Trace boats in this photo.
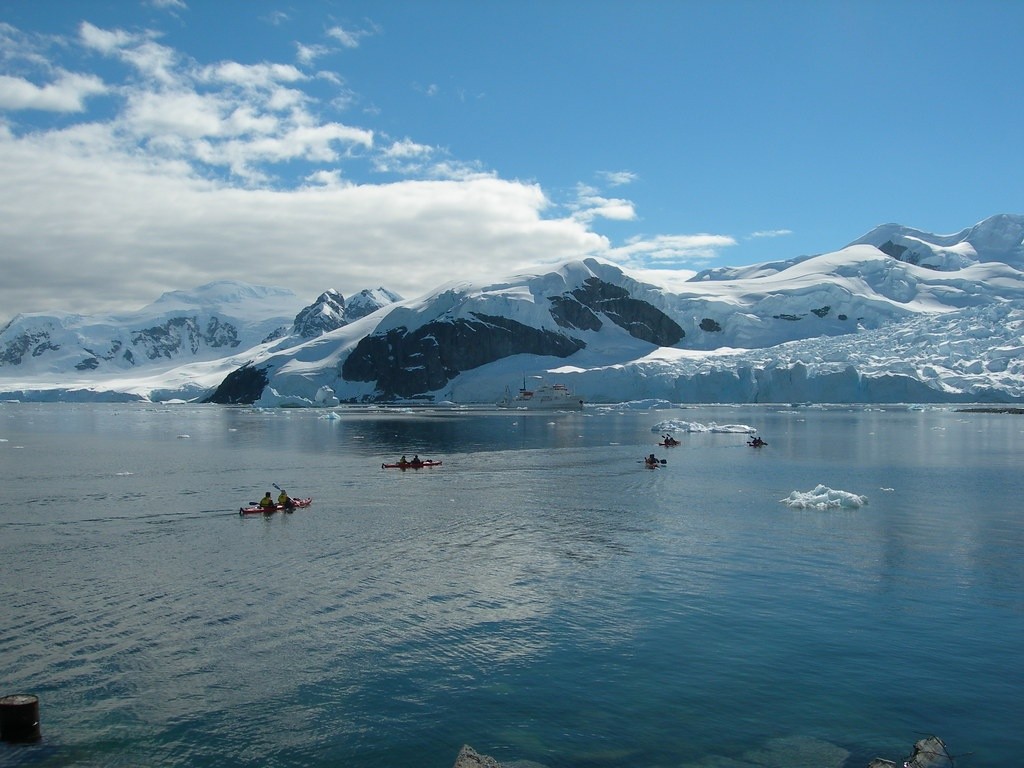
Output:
[383,460,443,469]
[749,440,766,446]
[645,457,657,470]
[240,498,312,513]
[496,373,583,411]
[660,439,680,446]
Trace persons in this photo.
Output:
[414,455,420,461]
[400,456,408,464]
[664,437,675,444]
[278,490,289,504]
[260,492,273,506]
[752,437,763,443]
[645,454,659,463]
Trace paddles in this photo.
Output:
[667,433,676,443]
[661,435,665,438]
[248,502,260,505]
[272,482,300,507]
[750,435,768,445]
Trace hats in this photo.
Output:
[415,455,418,457]
[402,456,406,458]
